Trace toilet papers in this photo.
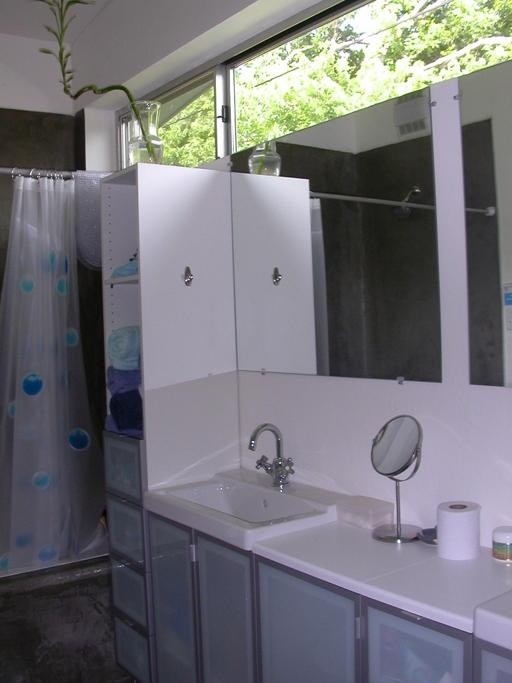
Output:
[436,499,482,561]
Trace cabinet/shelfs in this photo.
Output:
[235,171,317,382]
[253,558,472,683]
[141,512,254,683]
[98,163,230,683]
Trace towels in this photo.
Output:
[106,323,144,437]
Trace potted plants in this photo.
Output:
[39,0,165,163]
[249,139,281,176]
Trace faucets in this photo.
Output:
[248,422,295,487]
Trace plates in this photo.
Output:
[416,526,436,546]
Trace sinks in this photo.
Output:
[141,475,339,553]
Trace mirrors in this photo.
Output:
[371,412,429,544]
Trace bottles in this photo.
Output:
[491,525,511,563]
